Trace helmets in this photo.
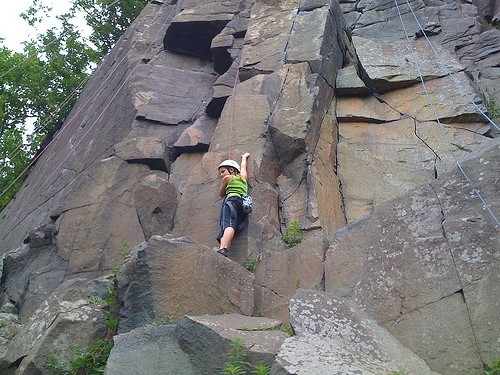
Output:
[218,159,240,173]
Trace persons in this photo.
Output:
[211,152,250,258]
[26,120,64,170]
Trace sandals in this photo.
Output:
[216,248,228,258]
